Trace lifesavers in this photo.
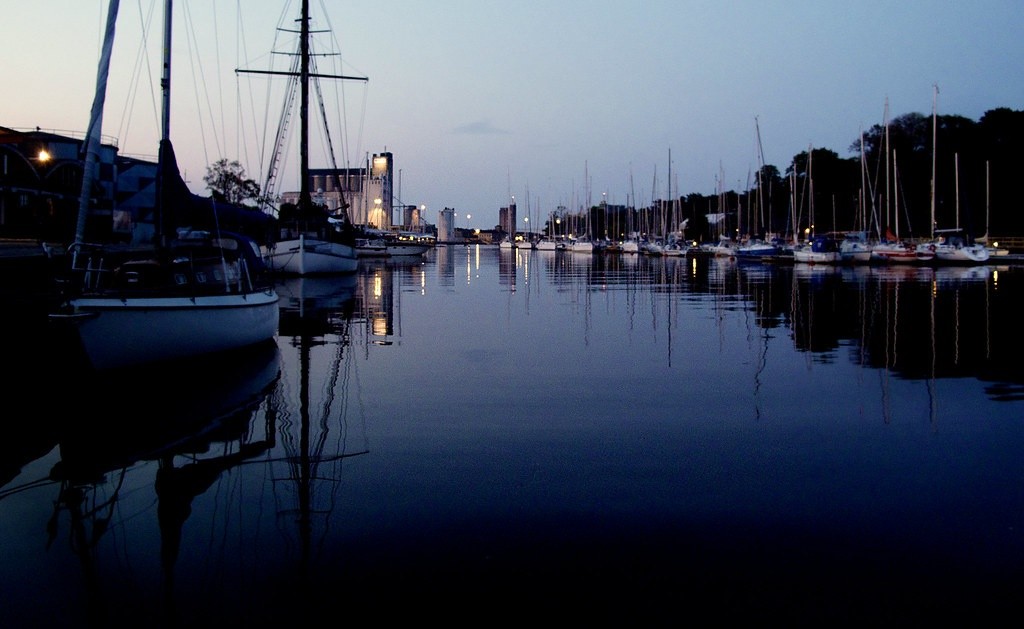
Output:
[909,243,917,251]
[929,244,937,252]
[46,251,87,287]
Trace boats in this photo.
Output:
[22,335,288,629]
[271,271,363,629]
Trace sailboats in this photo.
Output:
[497,82,1010,266]
[1,0,440,374]
